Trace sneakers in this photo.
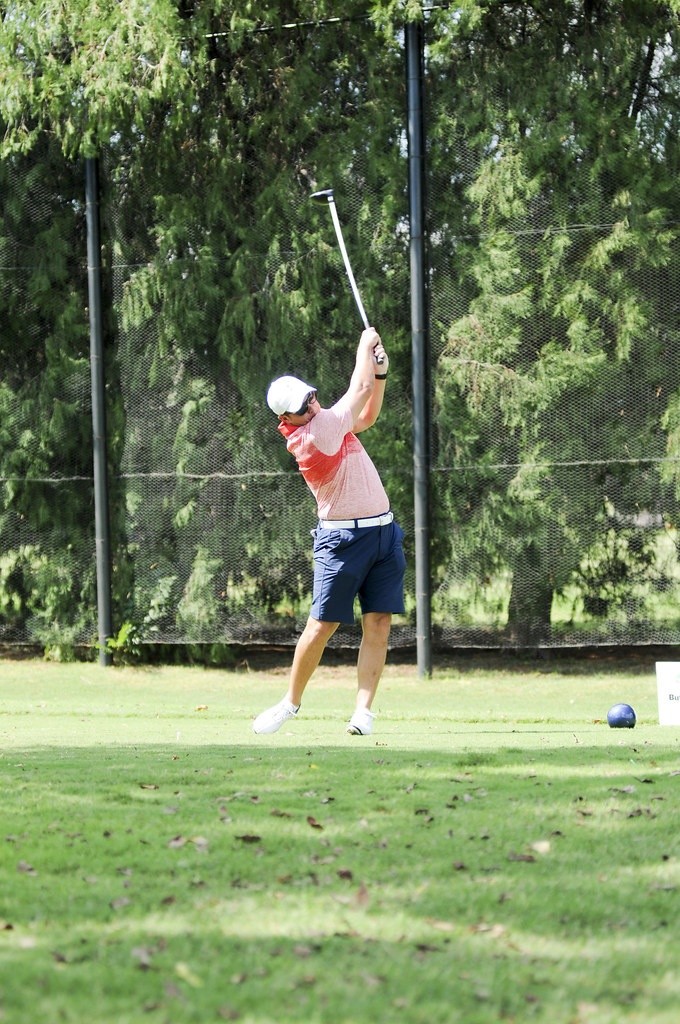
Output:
[251,692,301,734]
[345,706,376,735]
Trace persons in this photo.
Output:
[255,325,407,737]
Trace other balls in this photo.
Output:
[607,704,635,729]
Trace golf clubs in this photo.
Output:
[307,189,385,366]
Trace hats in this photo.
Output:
[267,375,318,416]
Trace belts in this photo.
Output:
[320,512,394,529]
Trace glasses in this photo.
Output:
[285,391,317,416]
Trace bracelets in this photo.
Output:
[376,372,388,379]
